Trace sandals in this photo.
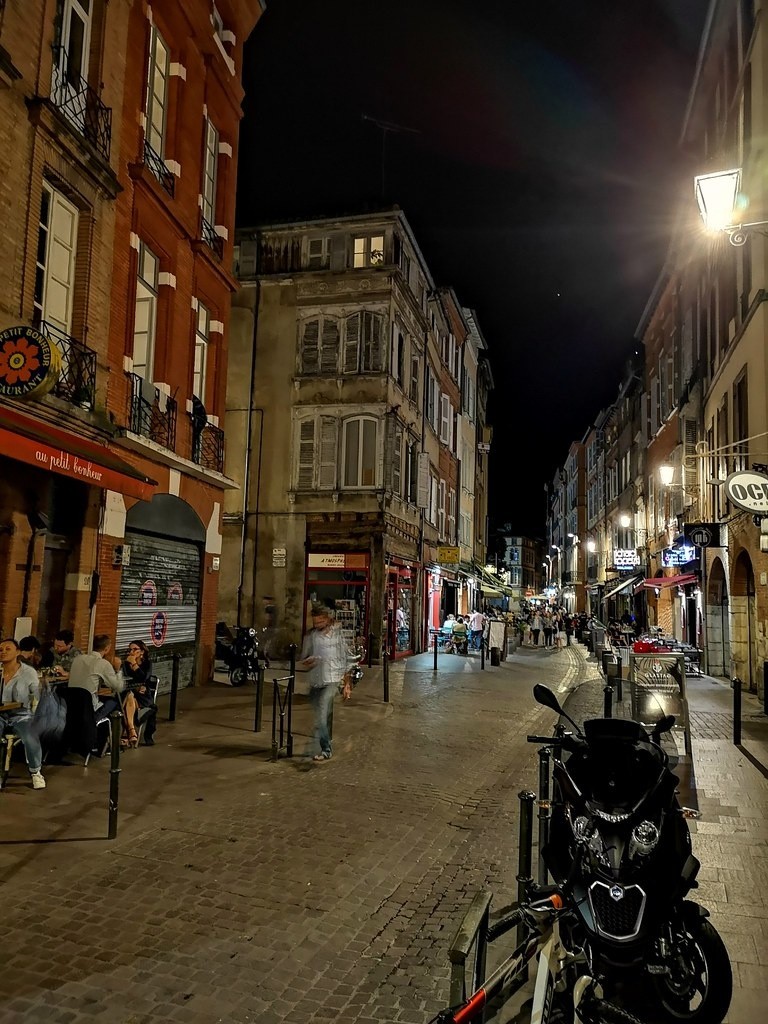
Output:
[119,729,137,750]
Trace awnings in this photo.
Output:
[644,575,697,589]
[603,577,638,599]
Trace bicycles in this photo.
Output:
[425,871,651,1024]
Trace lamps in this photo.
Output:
[688,152,768,248]
[658,458,701,498]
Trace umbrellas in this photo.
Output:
[482,586,503,598]
[529,596,548,610]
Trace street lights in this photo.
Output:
[551,544,561,590]
[543,563,548,586]
[568,533,578,582]
[546,555,552,584]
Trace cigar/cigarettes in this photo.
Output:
[348,697,350,698]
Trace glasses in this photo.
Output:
[126,648,142,652]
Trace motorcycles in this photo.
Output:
[526,678,733,1024]
[214,622,267,688]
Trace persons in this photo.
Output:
[527,601,574,652]
[0,629,153,789]
[443,606,505,654]
[300,607,352,761]
[396,606,406,627]
[572,610,597,644]
[607,608,637,648]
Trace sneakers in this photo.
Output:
[31,772,46,789]
[314,746,333,760]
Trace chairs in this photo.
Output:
[42,687,112,766]
[429,628,471,654]
[1,734,20,788]
[134,675,159,750]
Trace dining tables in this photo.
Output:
[98,685,113,696]
[44,675,68,684]
[0,699,23,711]
